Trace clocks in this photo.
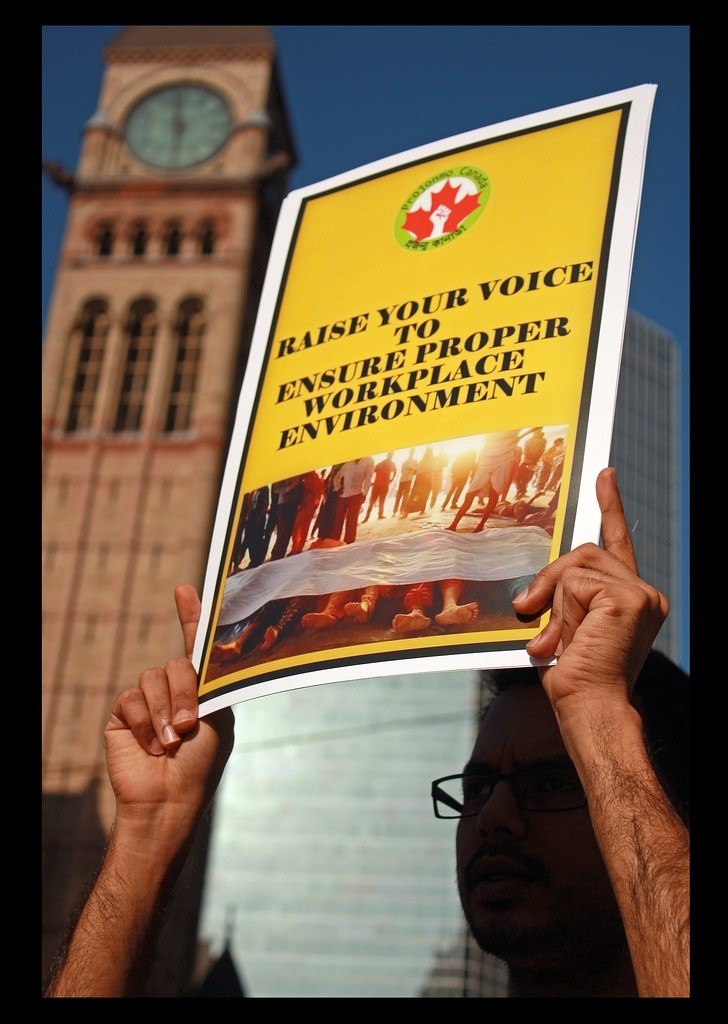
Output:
[120,83,233,170]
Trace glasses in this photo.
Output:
[432,758,587,818]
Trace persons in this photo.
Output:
[41,467,691,998]
[209,428,565,660]
